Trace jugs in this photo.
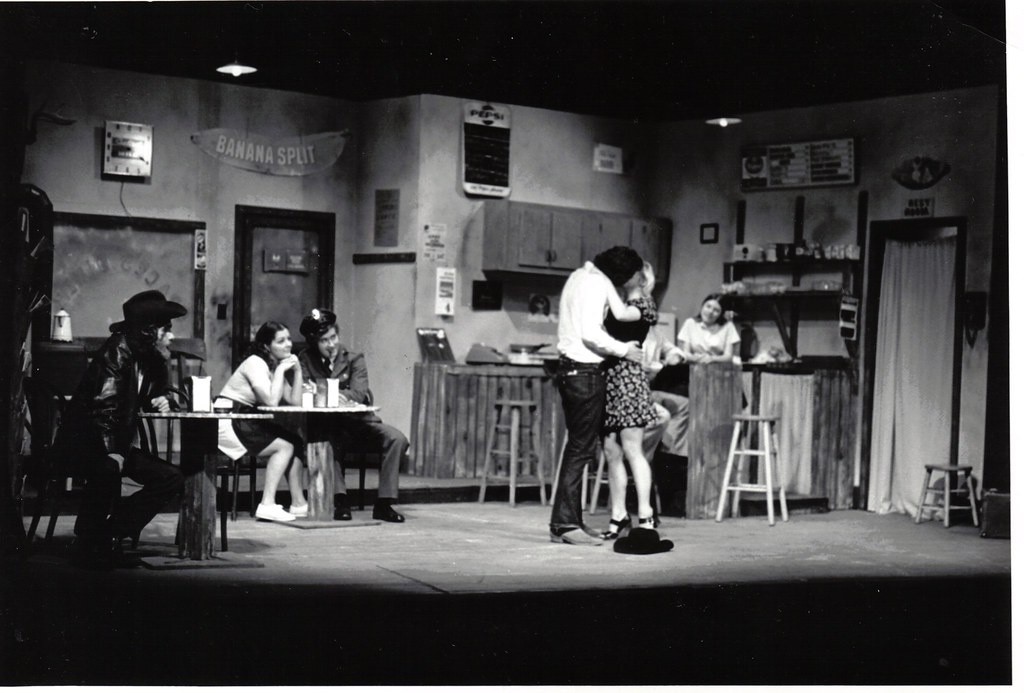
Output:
[52,308,73,343]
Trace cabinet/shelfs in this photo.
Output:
[465,197,674,282]
[407,373,566,485]
[734,365,859,510]
[719,257,860,357]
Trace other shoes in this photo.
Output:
[551,523,605,546]
[67,538,133,570]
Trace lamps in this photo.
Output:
[215,49,258,78]
[704,108,743,129]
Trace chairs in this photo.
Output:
[136,386,230,553]
[183,376,258,520]
[21,373,126,560]
[340,390,379,512]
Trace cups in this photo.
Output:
[316,378,325,407]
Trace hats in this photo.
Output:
[614,527,675,554]
[299,308,339,337]
[110,290,188,332]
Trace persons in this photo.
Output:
[46,289,188,571]
[279,309,409,524]
[548,245,690,548]
[213,320,309,523]
[677,293,748,410]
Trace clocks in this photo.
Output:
[101,117,154,178]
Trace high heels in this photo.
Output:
[638,508,662,528]
[600,512,633,541]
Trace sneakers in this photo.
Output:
[289,501,308,517]
[256,502,295,523]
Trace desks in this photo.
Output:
[256,404,383,530]
[136,411,275,571]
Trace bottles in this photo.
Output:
[301,384,314,408]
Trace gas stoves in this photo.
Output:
[509,351,558,364]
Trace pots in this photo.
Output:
[510,343,551,352]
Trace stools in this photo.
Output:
[477,397,547,508]
[715,412,791,528]
[915,461,979,529]
[588,431,662,517]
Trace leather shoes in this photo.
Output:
[334,504,351,519]
[372,506,404,522]
